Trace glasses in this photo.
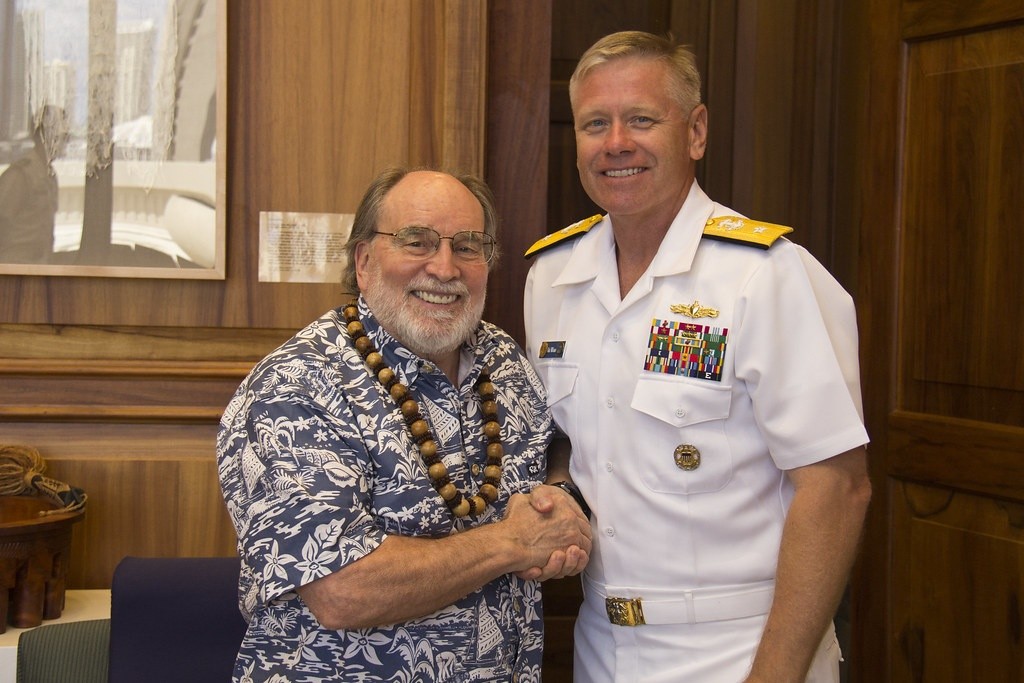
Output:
[368,226,497,264]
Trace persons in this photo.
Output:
[216,165,595,683]
[522,30,875,683]
[0,105,70,265]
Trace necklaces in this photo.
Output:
[343,299,504,516]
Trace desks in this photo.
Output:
[0,492,87,635]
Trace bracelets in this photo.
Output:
[550,480,592,522]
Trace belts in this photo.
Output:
[581,577,775,627]
